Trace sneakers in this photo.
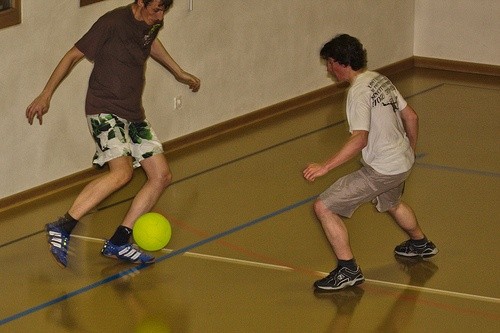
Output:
[394,239,438,256]
[101,239,156,264]
[45,216,70,268]
[314,264,365,290]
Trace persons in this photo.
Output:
[26,0,201,268]
[302,33,439,290]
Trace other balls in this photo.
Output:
[134,213,170,250]
